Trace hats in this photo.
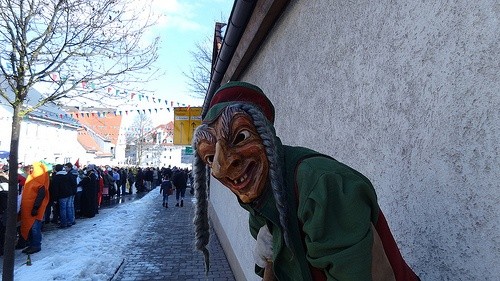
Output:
[201,81,275,125]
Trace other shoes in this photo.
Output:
[162,203,168,208]
[175,203,179,207]
[181,200,183,207]
[22,246,41,254]
[44,215,76,228]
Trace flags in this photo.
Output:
[74,159,81,168]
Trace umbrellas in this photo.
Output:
[0,151,10,159]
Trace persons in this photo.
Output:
[194,81,423,281]
[160,175,172,208]
[172,169,188,207]
[0,161,188,255]
